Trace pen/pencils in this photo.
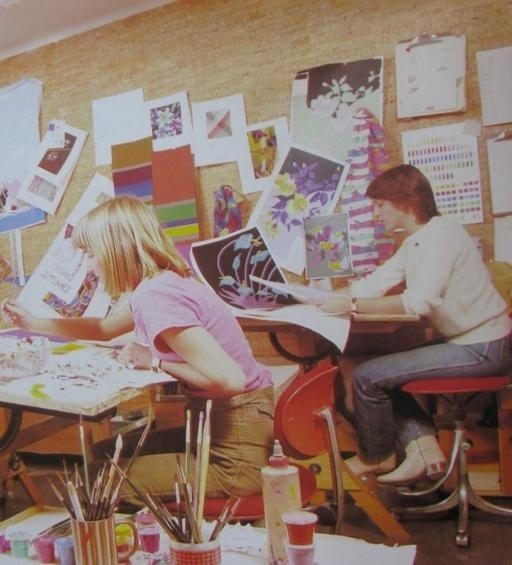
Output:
[3,291,13,310]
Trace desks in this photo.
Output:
[1,507,419,565]
[1,327,160,507]
[232,312,420,544]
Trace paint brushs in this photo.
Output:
[46,409,152,520]
[104,400,241,544]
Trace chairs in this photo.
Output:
[390,374,509,544]
[166,363,343,533]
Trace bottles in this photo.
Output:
[259,438,302,565]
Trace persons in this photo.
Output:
[2,195,280,515]
[287,163,510,487]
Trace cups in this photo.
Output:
[68,510,139,565]
[53,538,75,565]
[32,535,56,563]
[167,528,223,565]
[280,511,318,545]
[284,539,316,565]
[134,513,161,553]
[9,532,30,558]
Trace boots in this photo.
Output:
[378,435,446,484]
[344,452,396,476]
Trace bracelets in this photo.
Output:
[351,297,359,313]
